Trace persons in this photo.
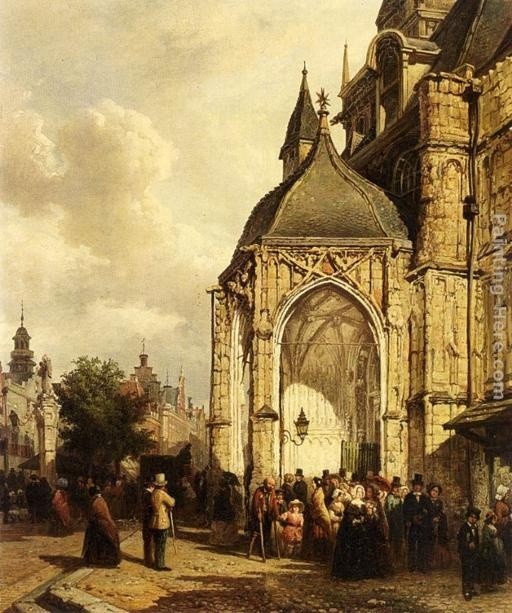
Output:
[0,444,512,602]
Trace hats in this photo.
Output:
[426,481,441,495]
[287,497,305,512]
[464,507,482,521]
[412,473,424,486]
[151,470,168,486]
[390,474,405,488]
[294,467,386,486]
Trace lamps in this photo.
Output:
[278,406,310,445]
